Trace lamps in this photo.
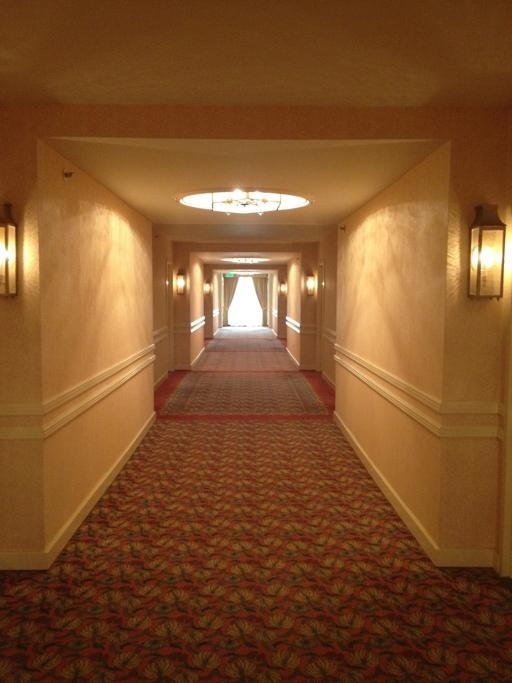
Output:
[175,267,186,295]
[465,201,506,299]
[173,187,313,214]
[204,279,211,295]
[306,272,317,296]
[280,281,286,294]
[0,201,20,298]
